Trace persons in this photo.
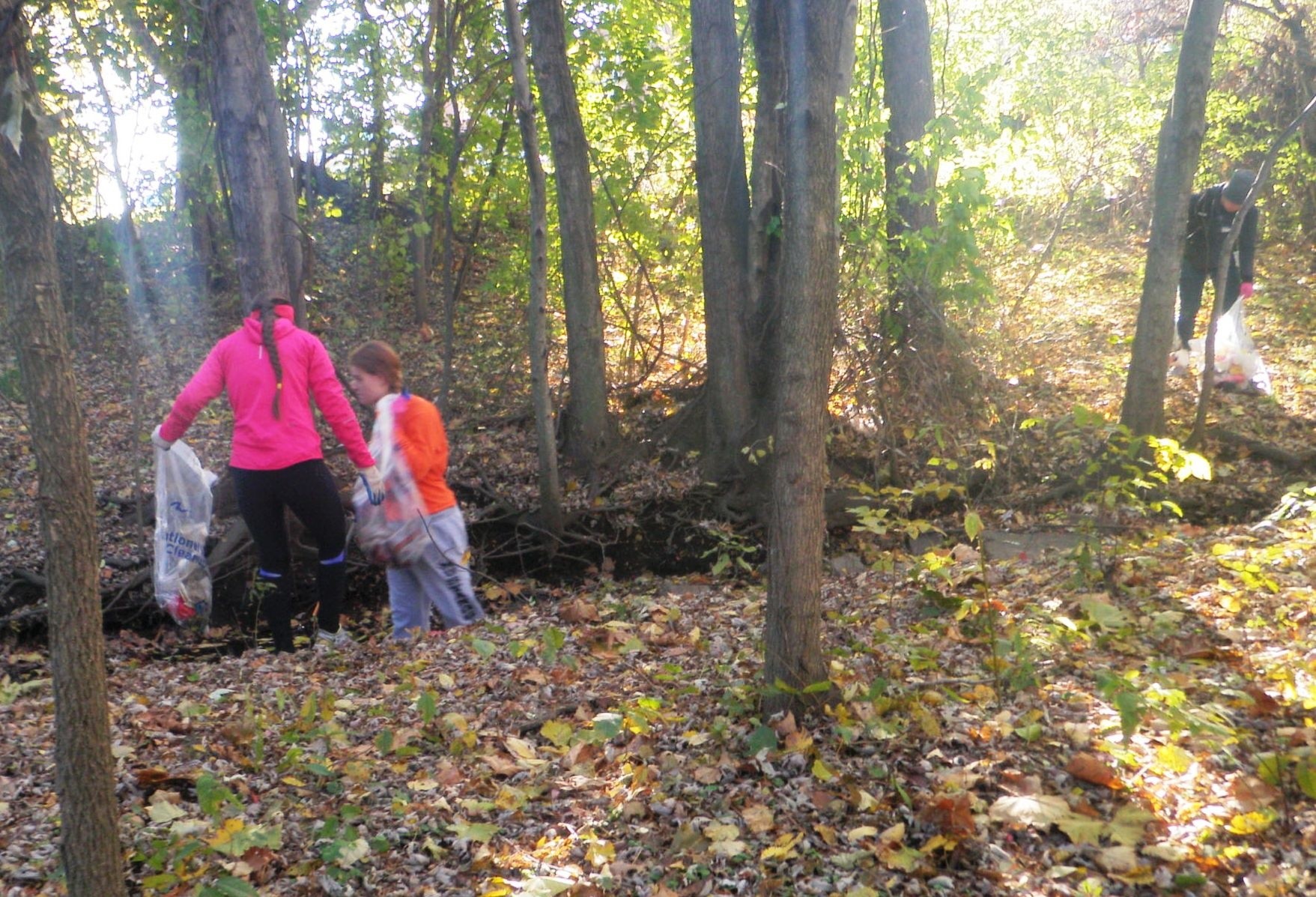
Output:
[151,288,386,657]
[346,339,487,642]
[1177,170,1258,353]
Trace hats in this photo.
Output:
[1223,166,1255,204]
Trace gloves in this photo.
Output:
[354,465,385,505]
[151,424,176,451]
[1239,280,1253,298]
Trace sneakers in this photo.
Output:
[313,622,359,652]
